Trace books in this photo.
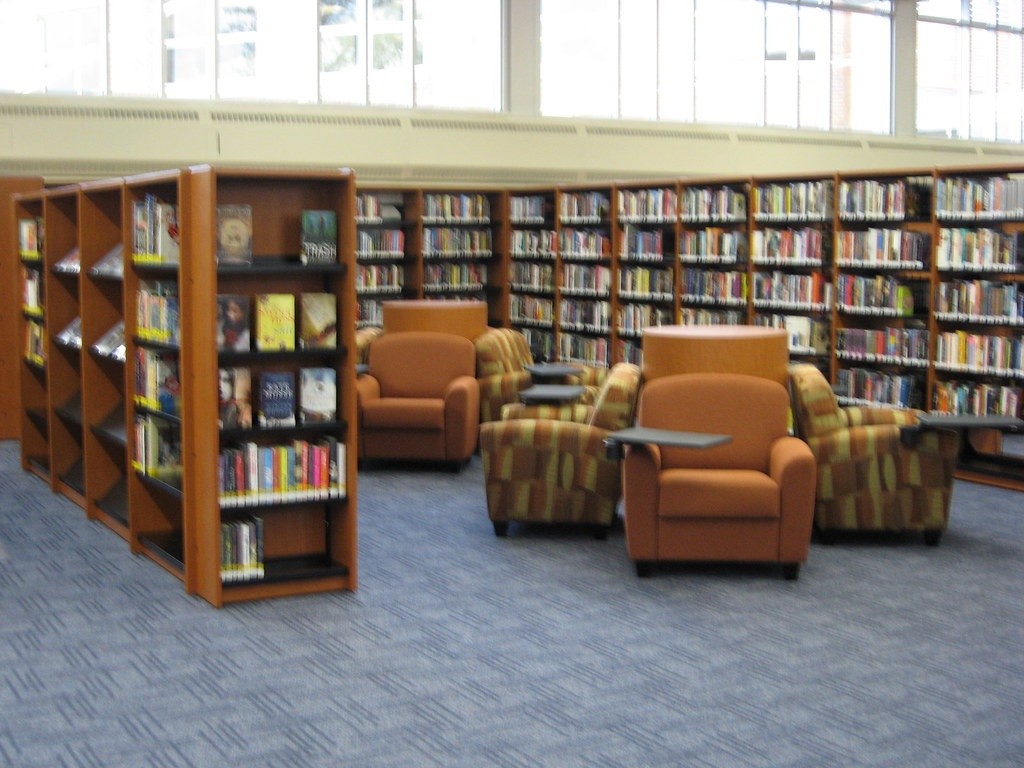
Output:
[257,372,296,428]
[216,294,251,351]
[217,367,253,431]
[300,209,338,263]
[299,292,337,350]
[55,244,125,362]
[220,437,346,583]
[215,204,252,265]
[132,196,183,477]
[255,293,296,352]
[356,172,1024,417]
[19,217,44,364]
[299,368,336,425]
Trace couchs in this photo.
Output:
[478,362,643,540]
[475,325,607,423]
[789,361,961,549]
[602,370,819,582]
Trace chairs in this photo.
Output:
[356,330,481,476]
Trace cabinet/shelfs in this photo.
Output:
[509,162,1024,490]
[0,165,359,608]
[355,182,510,334]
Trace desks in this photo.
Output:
[383,301,491,337]
[642,324,790,384]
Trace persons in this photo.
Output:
[219,297,250,349]
[218,369,242,430]
